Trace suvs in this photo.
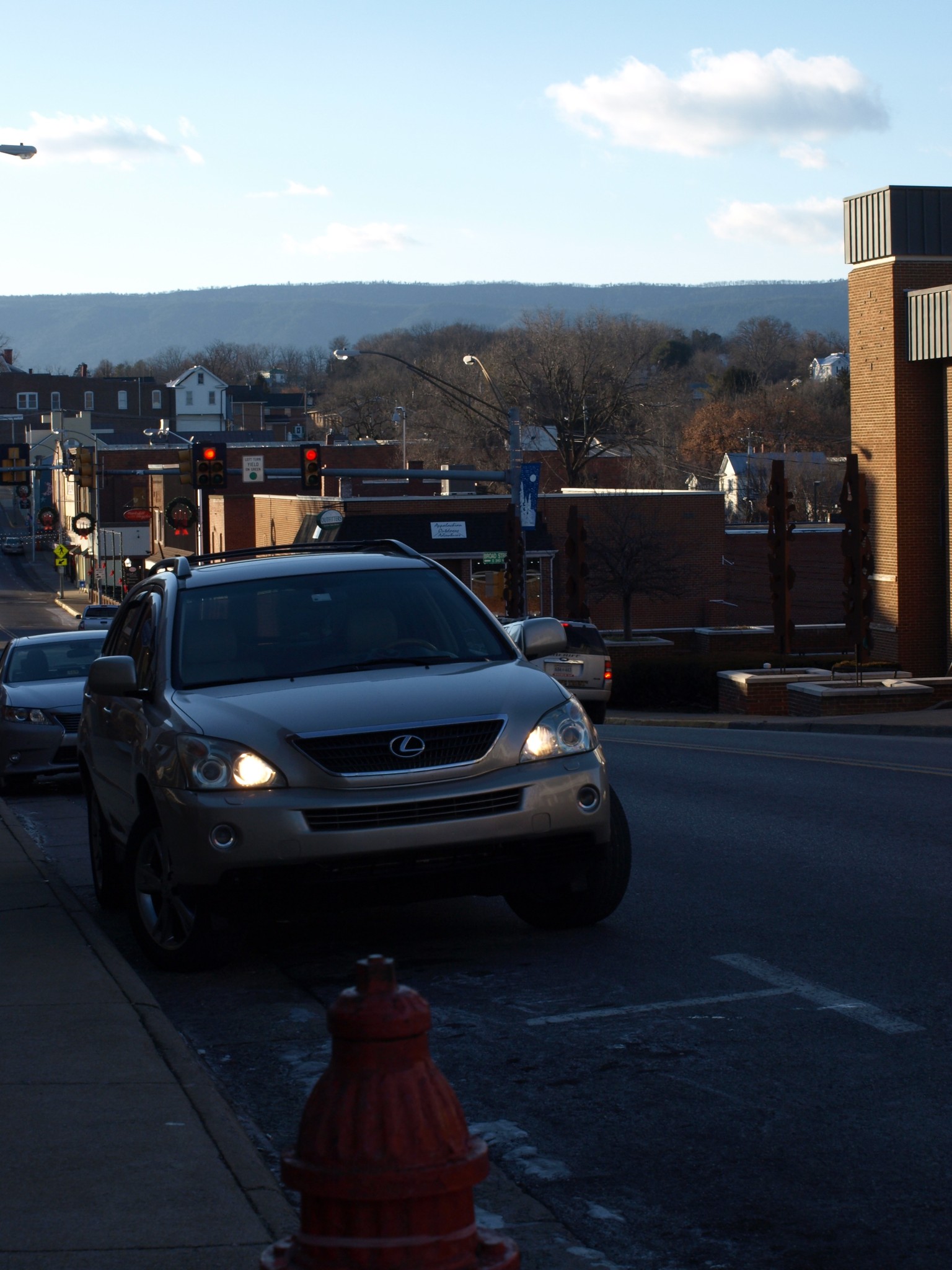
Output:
[502,618,613,727]
[68,533,631,983]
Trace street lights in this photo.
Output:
[814,479,821,521]
[392,402,408,476]
[332,346,524,613]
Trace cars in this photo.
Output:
[1,536,24,555]
[0,630,116,796]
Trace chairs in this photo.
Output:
[181,616,269,686]
[328,604,405,666]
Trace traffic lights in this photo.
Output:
[191,440,229,491]
[73,451,93,487]
[301,442,322,489]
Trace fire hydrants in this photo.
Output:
[252,950,528,1270]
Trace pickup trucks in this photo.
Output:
[75,604,121,632]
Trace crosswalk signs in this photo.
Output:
[54,543,68,559]
